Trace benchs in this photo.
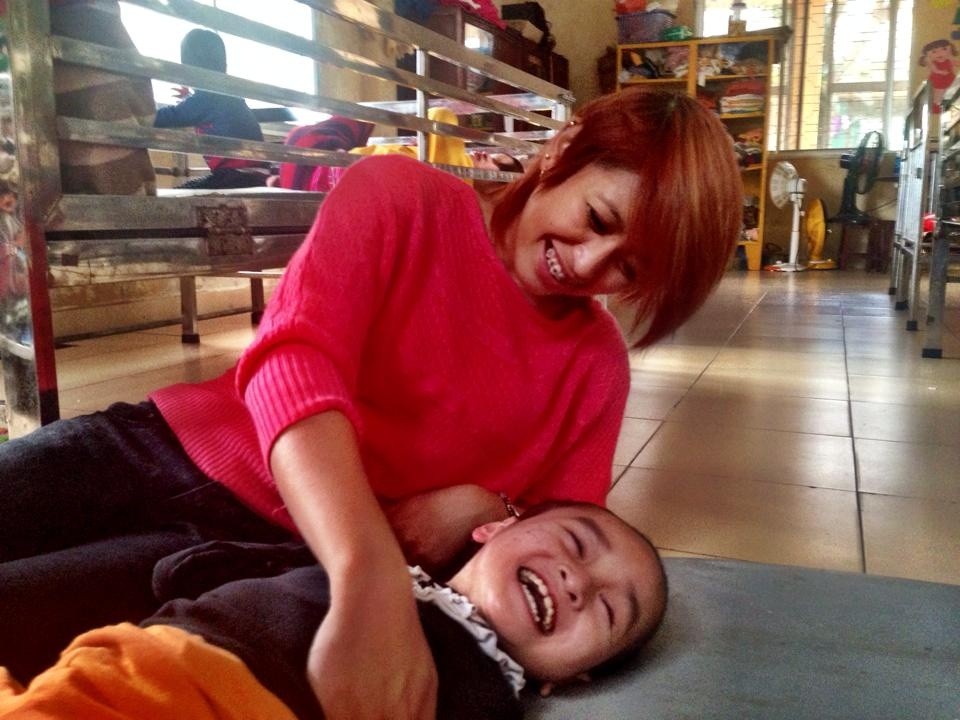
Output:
[0,0,577,427]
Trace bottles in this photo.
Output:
[728,0,747,37]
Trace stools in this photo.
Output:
[838,220,897,273]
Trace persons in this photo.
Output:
[0,0,156,194]
[0,86,745,690]
[0,499,671,719]
[154,29,269,189]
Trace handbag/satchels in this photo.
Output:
[501,2,552,47]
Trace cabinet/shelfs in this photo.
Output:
[617,31,781,271]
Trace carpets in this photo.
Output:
[528,556,960,720]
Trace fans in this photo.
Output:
[828,131,884,224]
[762,158,811,271]
[802,198,837,270]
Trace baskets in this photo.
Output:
[615,9,678,45]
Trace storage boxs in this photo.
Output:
[614,10,677,44]
